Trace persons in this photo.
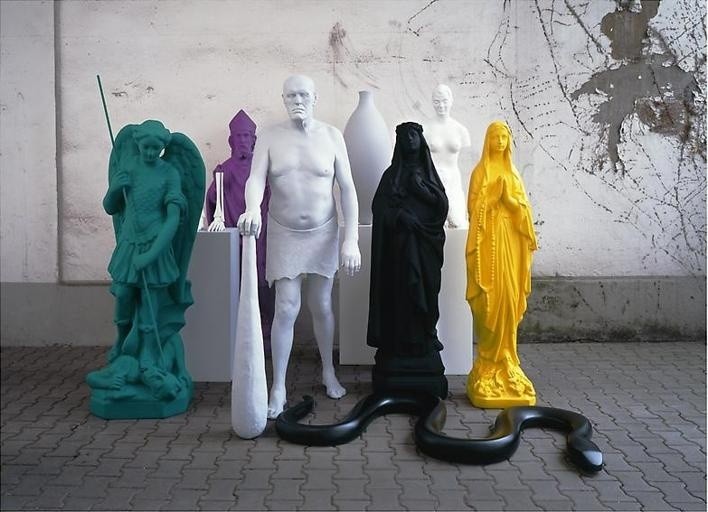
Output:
[461,119,540,410]
[423,82,464,228]
[84,119,207,385]
[235,73,364,422]
[205,108,278,358]
[80,353,183,402]
[366,120,450,389]
